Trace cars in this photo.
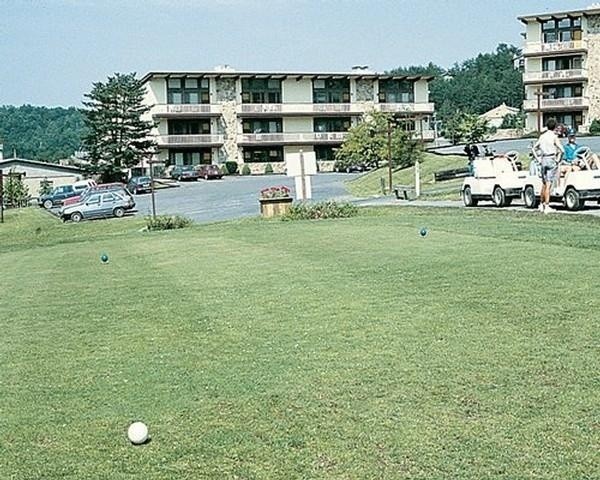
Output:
[333,155,364,173]
[34,161,226,224]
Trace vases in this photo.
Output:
[258,198,293,219]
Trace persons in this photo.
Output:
[531,119,564,215]
[560,129,578,164]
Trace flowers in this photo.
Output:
[261,184,290,198]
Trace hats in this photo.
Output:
[566,128,577,136]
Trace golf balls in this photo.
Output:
[126,421,148,444]
[420,230,427,235]
[102,255,108,262]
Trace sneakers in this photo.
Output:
[537,202,556,214]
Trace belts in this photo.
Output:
[542,153,555,157]
